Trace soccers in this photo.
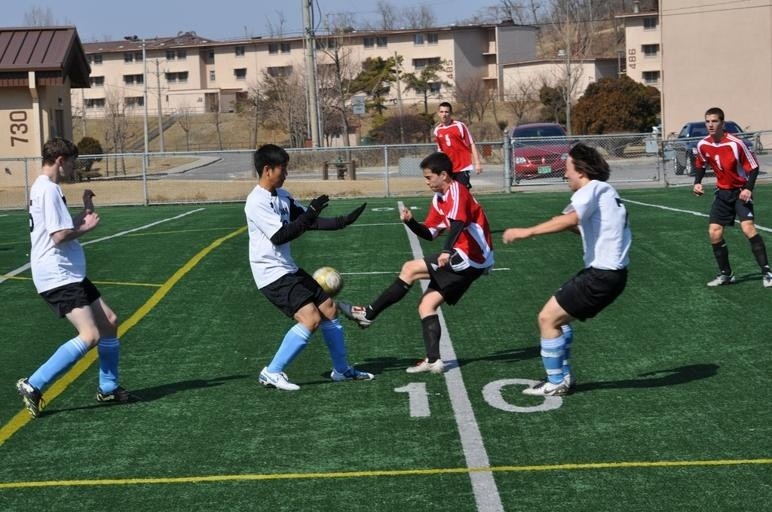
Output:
[313,266,342,297]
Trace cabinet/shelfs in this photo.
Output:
[323,160,355,180]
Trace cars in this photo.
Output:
[509,123,577,183]
[671,120,754,177]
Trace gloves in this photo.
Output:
[344,203,366,225]
[305,195,329,221]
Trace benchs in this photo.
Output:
[622,143,664,159]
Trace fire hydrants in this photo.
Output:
[334,157,348,181]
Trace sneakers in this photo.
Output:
[706,272,736,286]
[330,366,374,381]
[339,301,374,329]
[406,358,445,375]
[258,367,300,390]
[762,271,771,286]
[17,378,46,419]
[96,386,142,402]
[522,374,572,394]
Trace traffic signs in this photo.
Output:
[352,95,365,114]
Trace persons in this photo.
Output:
[337,153,497,375]
[14,138,143,418]
[244,144,375,391]
[500,143,634,397]
[693,106,772,288]
[432,101,483,191]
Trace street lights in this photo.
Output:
[557,49,573,137]
[122,32,147,161]
